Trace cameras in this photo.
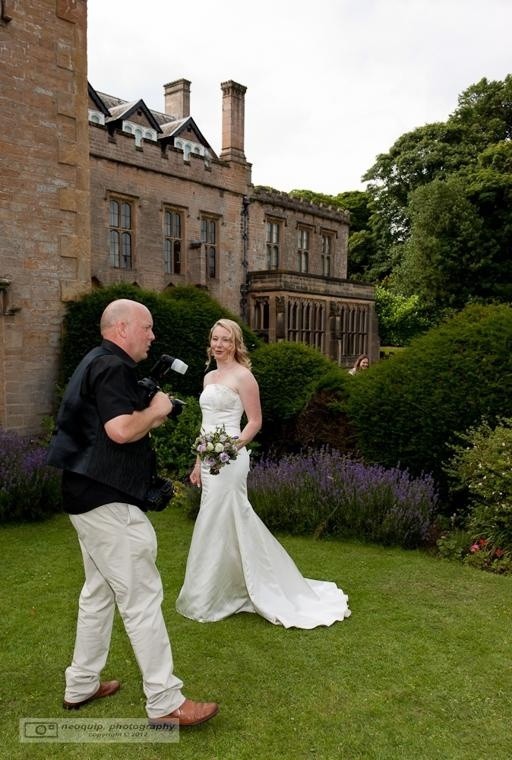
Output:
[138,353,189,421]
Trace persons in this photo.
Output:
[176,317,352,632]
[346,353,370,376]
[46,298,219,728]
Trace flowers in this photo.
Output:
[193,424,239,473]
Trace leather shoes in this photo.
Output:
[148,699,218,727]
[64,680,119,709]
